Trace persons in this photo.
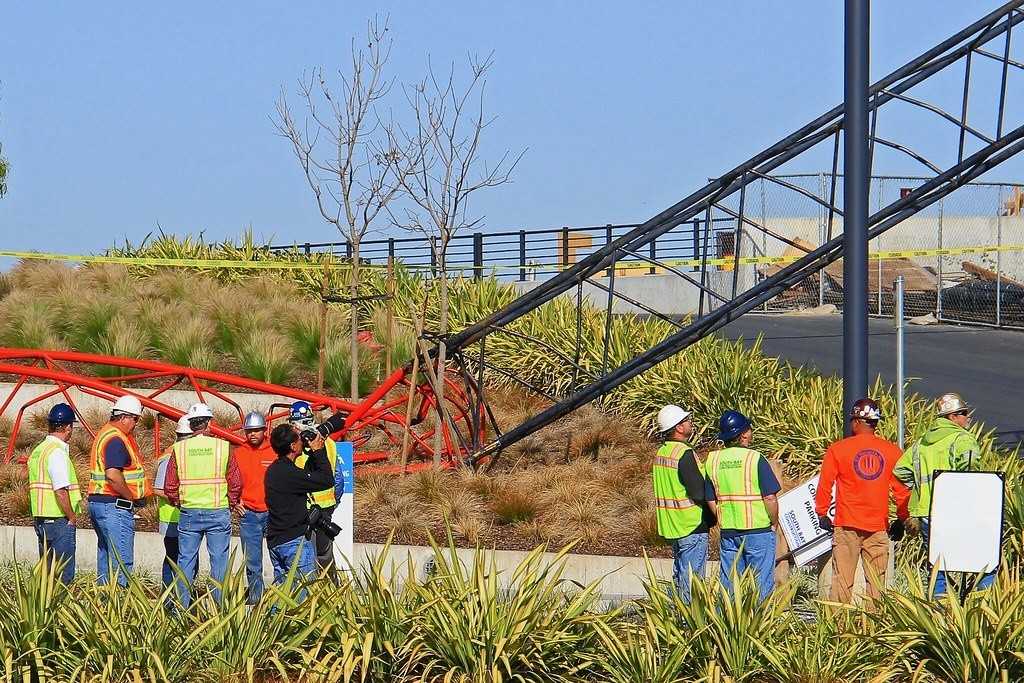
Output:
[234,412,278,618]
[27,403,82,601]
[286,402,344,589]
[705,411,782,626]
[892,394,997,619]
[652,404,717,624]
[263,424,336,632]
[815,398,913,636]
[153,414,198,612]
[164,404,243,618]
[87,394,153,607]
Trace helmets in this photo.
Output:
[243,412,266,429]
[175,415,194,433]
[934,393,969,415]
[657,405,691,432]
[286,401,314,420]
[110,395,142,416]
[45,403,79,423]
[850,398,882,419]
[717,411,751,439]
[187,403,213,420]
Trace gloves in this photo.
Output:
[888,519,904,541]
[819,516,834,532]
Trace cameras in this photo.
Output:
[300,413,346,448]
[306,504,343,541]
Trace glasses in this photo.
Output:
[850,418,858,422]
[953,411,968,417]
[245,428,263,433]
[126,414,138,423]
